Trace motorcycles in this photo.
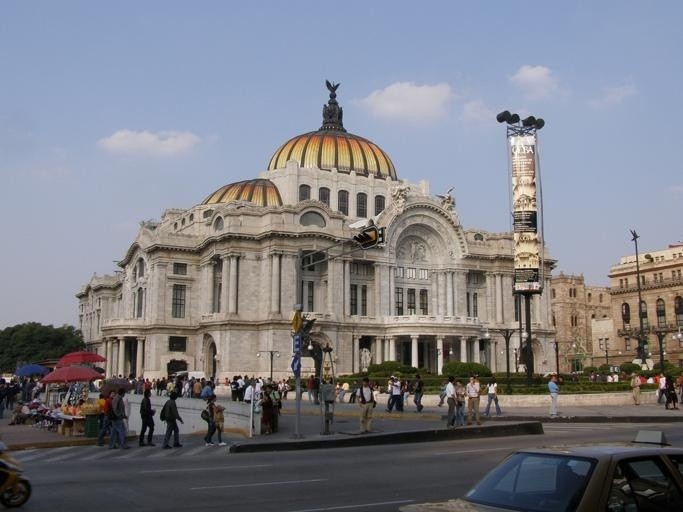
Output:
[0,440,31,507]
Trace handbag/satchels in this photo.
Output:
[201,406,210,422]
[159,403,166,421]
[275,398,282,409]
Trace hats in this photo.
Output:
[629,372,638,377]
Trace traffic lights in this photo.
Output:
[353,227,377,250]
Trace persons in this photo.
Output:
[547,375,560,416]
[571,370,683,410]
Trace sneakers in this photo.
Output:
[93,441,227,450]
[264,405,560,436]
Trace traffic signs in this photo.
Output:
[292,335,299,351]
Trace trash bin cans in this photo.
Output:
[610,366,618,372]
[85,414,99,438]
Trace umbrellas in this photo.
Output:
[14,364,49,377]
[55,351,106,369]
[39,367,105,385]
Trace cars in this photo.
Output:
[395,429,683,512]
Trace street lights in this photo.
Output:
[657,326,682,374]
[554,339,576,376]
[605,348,623,365]
[256,350,280,380]
[501,346,520,373]
[485,327,529,393]
